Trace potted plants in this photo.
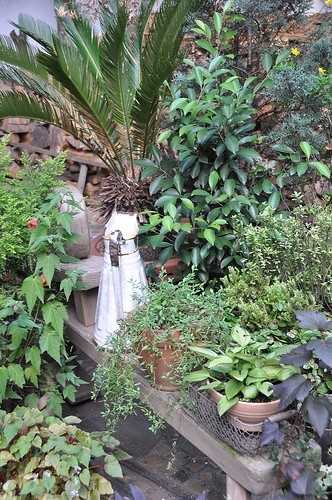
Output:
[183,328,301,436]
[89,265,224,444]
